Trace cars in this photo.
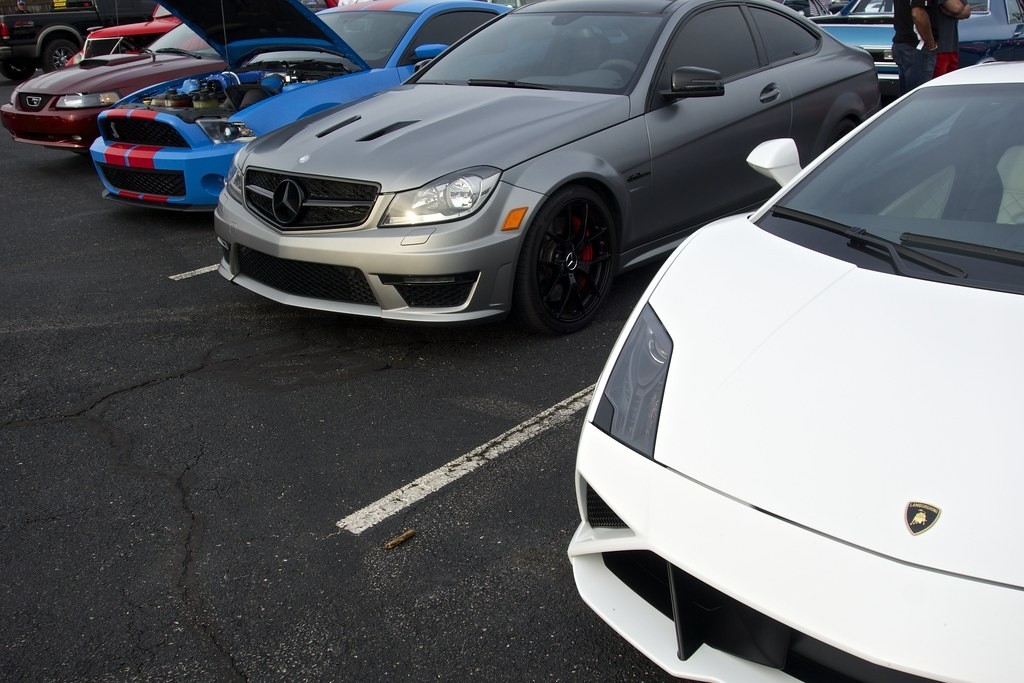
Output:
[567,60,1024,683]
[214,0,881,334]
[0,0,514,212]
[773,0,1024,103]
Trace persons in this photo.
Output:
[891,0,971,95]
[15,0,31,14]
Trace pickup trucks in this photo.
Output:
[0,1,158,81]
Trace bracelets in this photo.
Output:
[930,45,938,50]
